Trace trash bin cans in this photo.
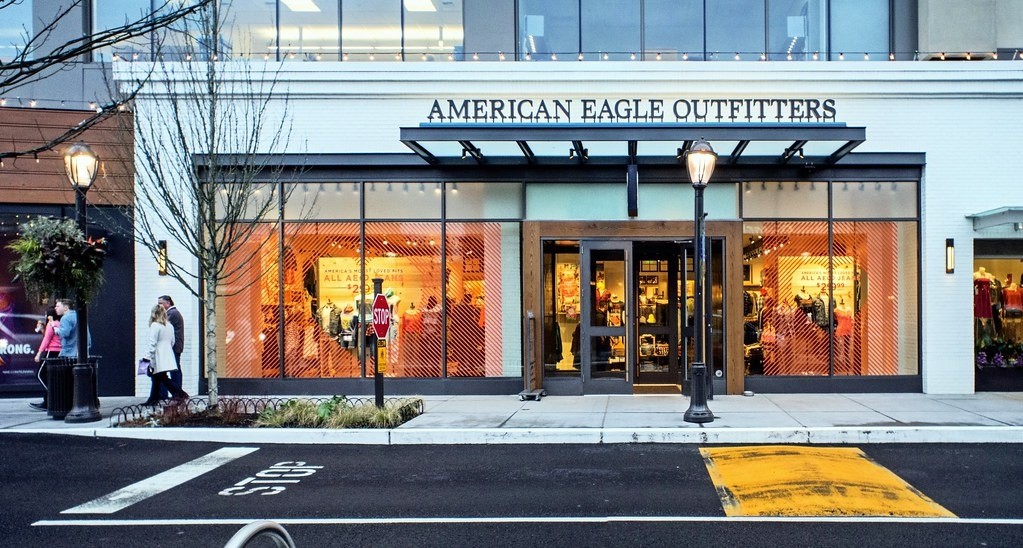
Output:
[41,355,102,420]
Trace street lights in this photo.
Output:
[62,139,104,424]
[682,137,719,423]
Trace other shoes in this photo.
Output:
[140,403,155,406]
[28,402,47,410]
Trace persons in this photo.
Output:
[774,301,795,375]
[384,292,401,364]
[420,296,443,377]
[313,301,342,377]
[355,295,375,362]
[833,302,852,367]
[1002,283,1023,309]
[28,298,93,412]
[138,295,190,405]
[813,291,834,349]
[401,309,422,377]
[973,267,995,328]
[987,275,1005,340]
[338,305,357,351]
[794,289,815,340]
[596,300,611,362]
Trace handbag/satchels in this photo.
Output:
[138,359,149,375]
[147,366,154,376]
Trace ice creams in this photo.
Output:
[48,315,53,322]
[38,320,42,324]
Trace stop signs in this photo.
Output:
[371,292,392,341]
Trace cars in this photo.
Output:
[0,312,49,380]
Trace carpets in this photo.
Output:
[632,386,681,394]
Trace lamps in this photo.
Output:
[158,240,167,276]
[945,238,955,273]
